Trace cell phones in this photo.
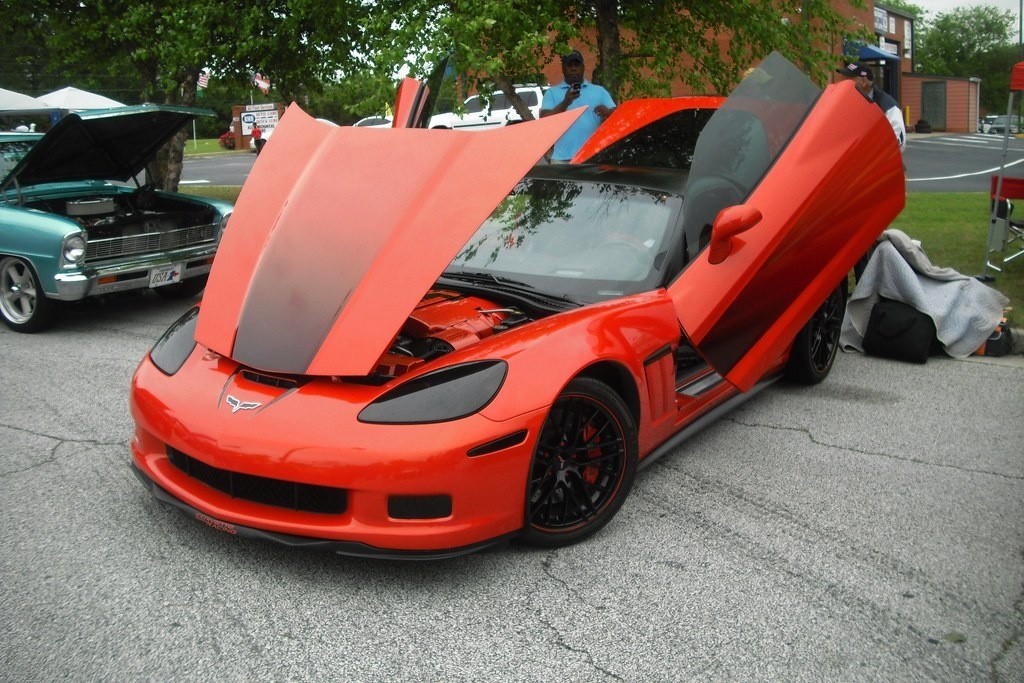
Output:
[573,84,581,90]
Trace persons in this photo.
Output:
[251,123,261,156]
[539,50,617,164]
[836,61,907,285]
[14,120,29,132]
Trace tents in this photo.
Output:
[0,83,127,117]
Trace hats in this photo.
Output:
[563,49,583,64]
[836,62,875,82]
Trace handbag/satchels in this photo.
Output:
[862,300,936,366]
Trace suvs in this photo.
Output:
[426,83,552,130]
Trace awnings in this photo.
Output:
[843,39,900,61]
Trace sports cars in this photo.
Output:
[129,44,907,561]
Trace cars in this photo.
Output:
[0,102,236,334]
[980,115,999,134]
[351,115,394,129]
[991,114,1024,135]
